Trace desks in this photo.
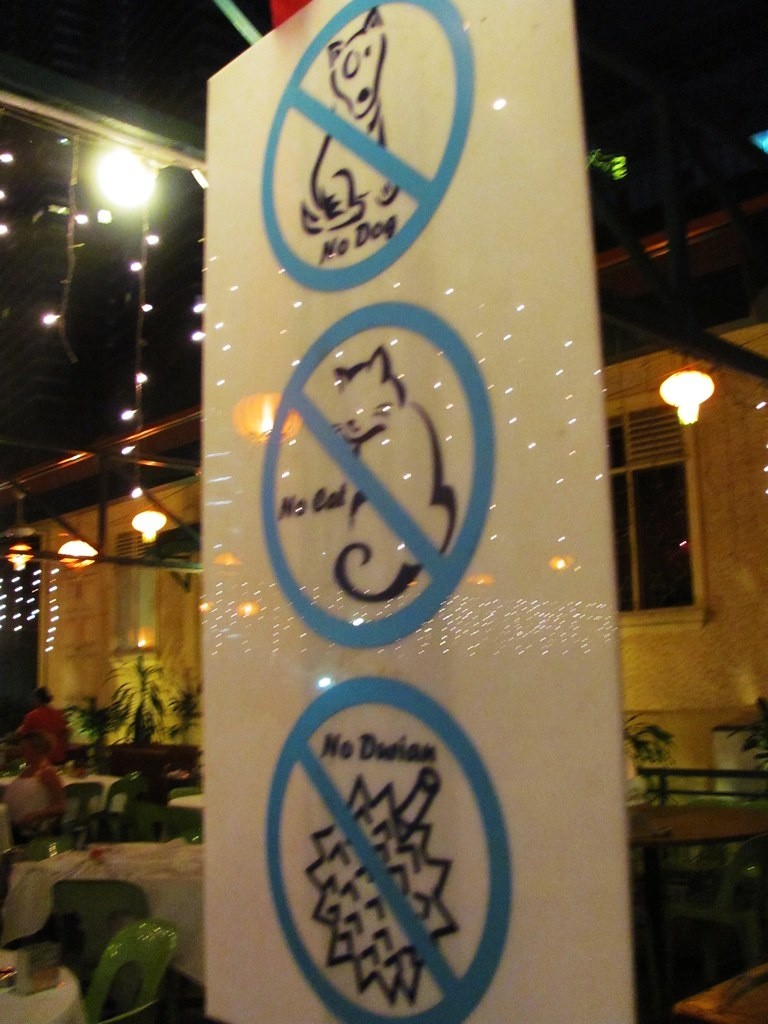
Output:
[0,843,204,1024]
[673,963,768,1024]
[627,804,768,1024]
[0,949,85,1024]
[0,774,128,832]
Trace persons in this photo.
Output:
[1,733,66,837]
[16,687,69,760]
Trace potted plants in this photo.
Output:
[64,696,127,766]
[107,656,174,799]
[167,683,203,772]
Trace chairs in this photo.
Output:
[85,917,177,1024]
[90,770,150,841]
[169,787,201,837]
[50,782,104,836]
[119,801,202,842]
[661,832,767,991]
[53,879,181,1024]
